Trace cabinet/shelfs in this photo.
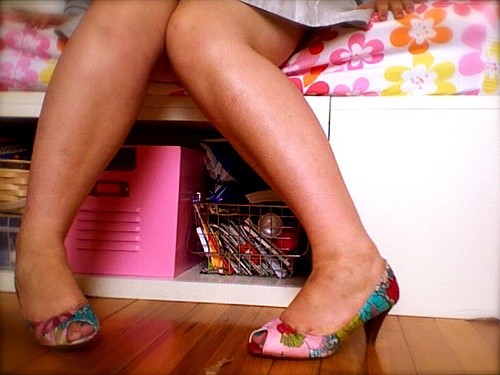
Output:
[0,89,500,323]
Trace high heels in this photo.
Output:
[247,260,400,360]
[14,279,101,349]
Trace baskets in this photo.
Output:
[0,159,31,214]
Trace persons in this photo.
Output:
[13,0,430,360]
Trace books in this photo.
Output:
[244,189,284,204]
[193,218,292,279]
